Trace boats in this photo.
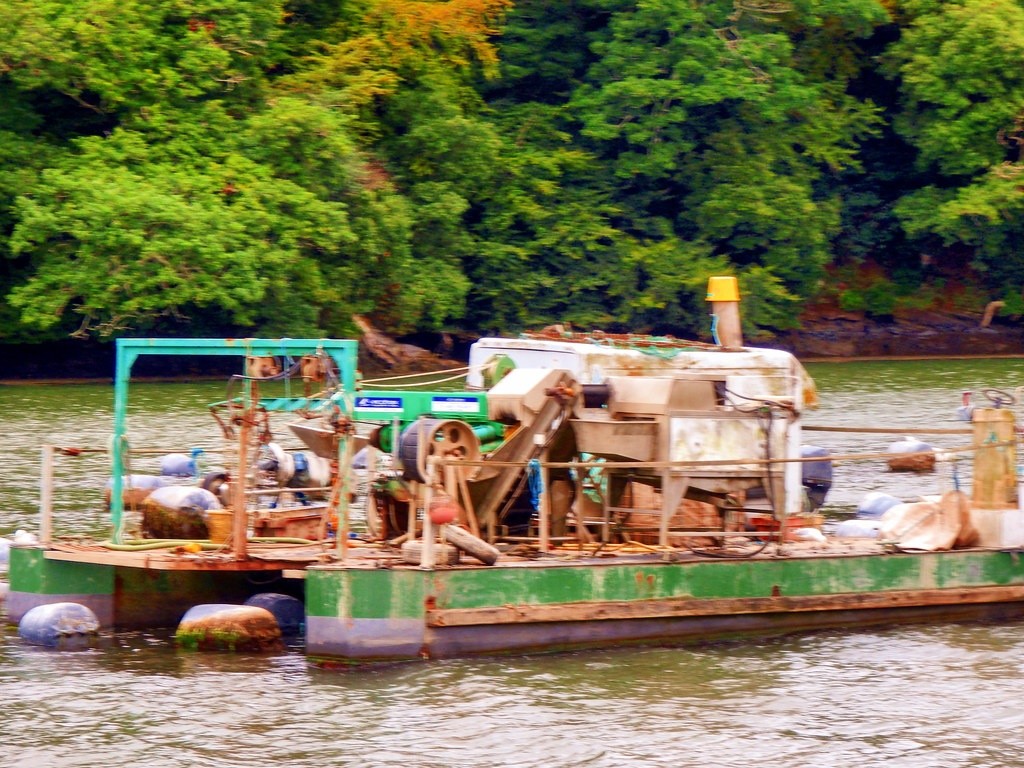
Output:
[5,277,1024,667]
